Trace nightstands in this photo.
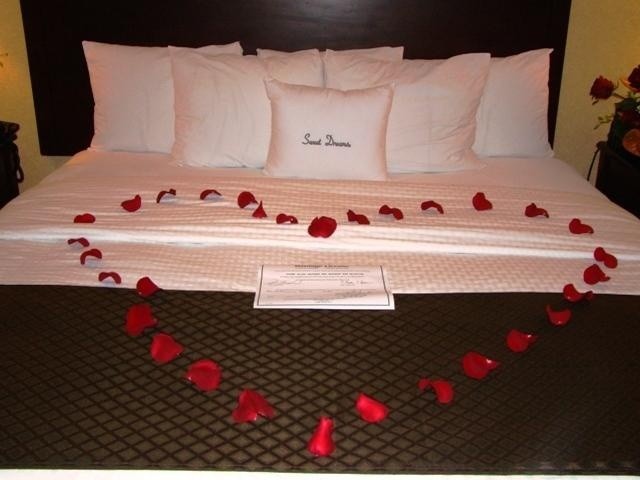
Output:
[595,141,639,217]
[0,121,22,207]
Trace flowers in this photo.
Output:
[587,67,640,164]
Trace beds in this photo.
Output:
[0,152,640,476]
